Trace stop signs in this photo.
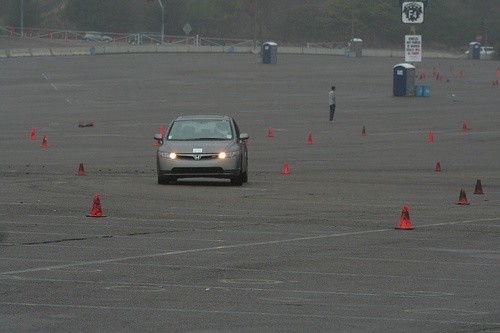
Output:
[476,34,482,42]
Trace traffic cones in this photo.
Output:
[427,131,434,142]
[157,126,165,145]
[394,205,415,230]
[266,127,274,137]
[455,187,471,205]
[472,179,484,195]
[76,162,86,176]
[305,131,313,144]
[29,128,36,140]
[40,133,48,148]
[461,121,468,132]
[78,121,93,127]
[85,192,107,217]
[434,161,442,172]
[280,161,291,174]
[360,124,368,136]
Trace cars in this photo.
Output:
[154,114,250,186]
[83,30,112,43]
[127,33,161,45]
[465,46,495,56]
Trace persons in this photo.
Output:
[328,84,336,122]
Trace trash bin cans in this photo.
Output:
[416,86,423,97]
[423,86,431,97]
